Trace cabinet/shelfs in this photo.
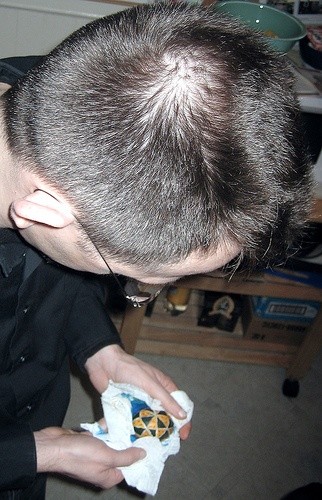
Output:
[105,261,321,369]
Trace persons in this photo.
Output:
[0,0,320,500]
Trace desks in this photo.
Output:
[268,47,322,168]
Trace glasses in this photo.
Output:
[33,187,167,310]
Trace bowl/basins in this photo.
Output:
[215,1,306,54]
